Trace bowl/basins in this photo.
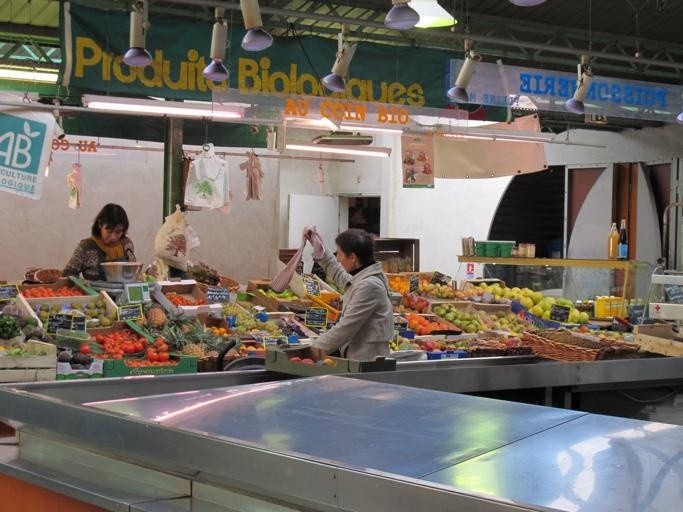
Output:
[100,261,143,285]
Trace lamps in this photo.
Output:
[276,138,393,162]
[321,23,357,93]
[565,56,594,114]
[239,0,273,52]
[80,93,251,123]
[202,7,231,82]
[383,1,457,30]
[123,0,154,68]
[447,39,482,101]
[443,127,606,152]
[286,114,407,135]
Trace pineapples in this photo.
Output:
[182,344,204,357]
[147,309,167,326]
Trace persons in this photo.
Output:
[64,204,139,283]
[301,228,395,362]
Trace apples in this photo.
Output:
[479,281,588,324]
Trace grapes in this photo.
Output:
[224,302,307,339]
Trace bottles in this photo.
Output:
[618,218,629,260]
[608,222,619,259]
[593,295,644,320]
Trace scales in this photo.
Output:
[86,281,151,304]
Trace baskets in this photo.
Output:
[522,330,642,365]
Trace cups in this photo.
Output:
[476,239,517,258]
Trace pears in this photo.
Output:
[431,303,521,334]
[30,300,117,326]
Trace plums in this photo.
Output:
[79,328,177,367]
